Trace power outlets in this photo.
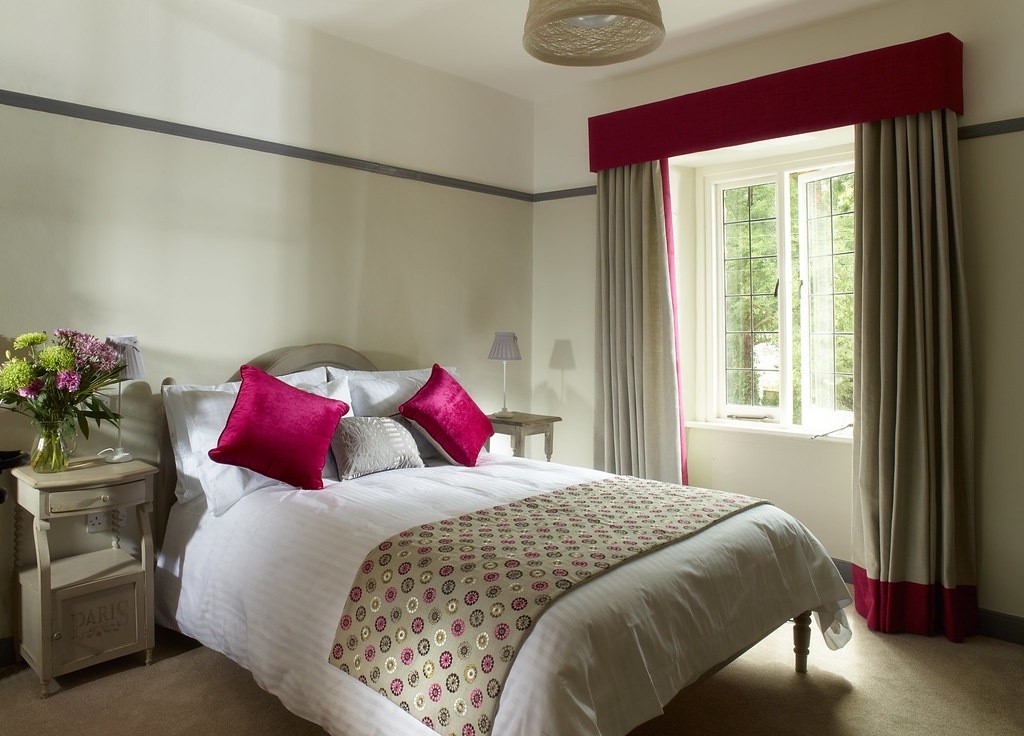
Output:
[84,508,128,535]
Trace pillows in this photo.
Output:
[181,375,355,516]
[399,363,495,467]
[161,366,328,505]
[207,363,351,491]
[330,412,429,482]
[326,365,458,460]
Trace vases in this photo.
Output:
[32,420,80,474]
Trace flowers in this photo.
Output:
[0,328,134,471]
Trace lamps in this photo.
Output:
[522,0,666,67]
[100,335,148,463]
[487,331,522,419]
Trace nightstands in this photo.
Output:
[8,452,161,698]
[483,410,562,462]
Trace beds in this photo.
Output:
[157,343,854,736]
